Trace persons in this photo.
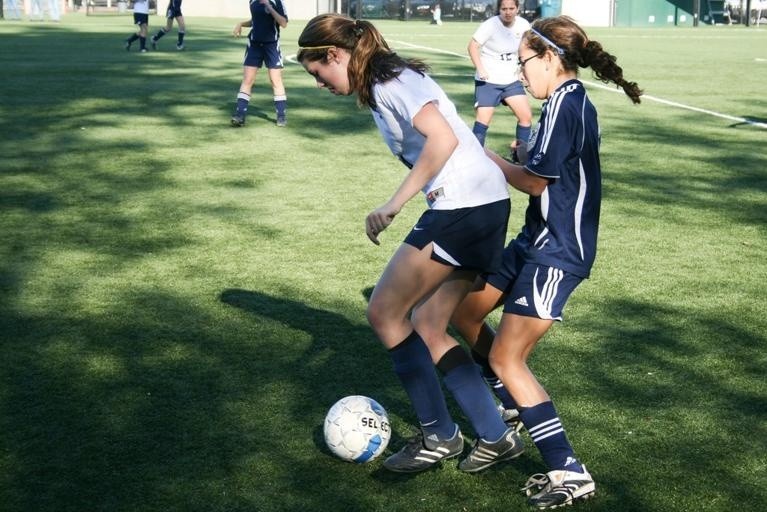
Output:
[230,0,289,128]
[125,0,156,53]
[430,3,441,25]
[450,15,645,511]
[469,0,533,163]
[297,15,527,477]
[150,0,185,51]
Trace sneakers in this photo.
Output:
[125,39,131,52]
[175,43,184,50]
[231,114,245,126]
[276,117,288,127]
[502,407,526,434]
[139,35,159,53]
[526,463,597,510]
[382,423,466,475]
[458,427,527,473]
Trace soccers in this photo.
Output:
[323,395,392,465]
